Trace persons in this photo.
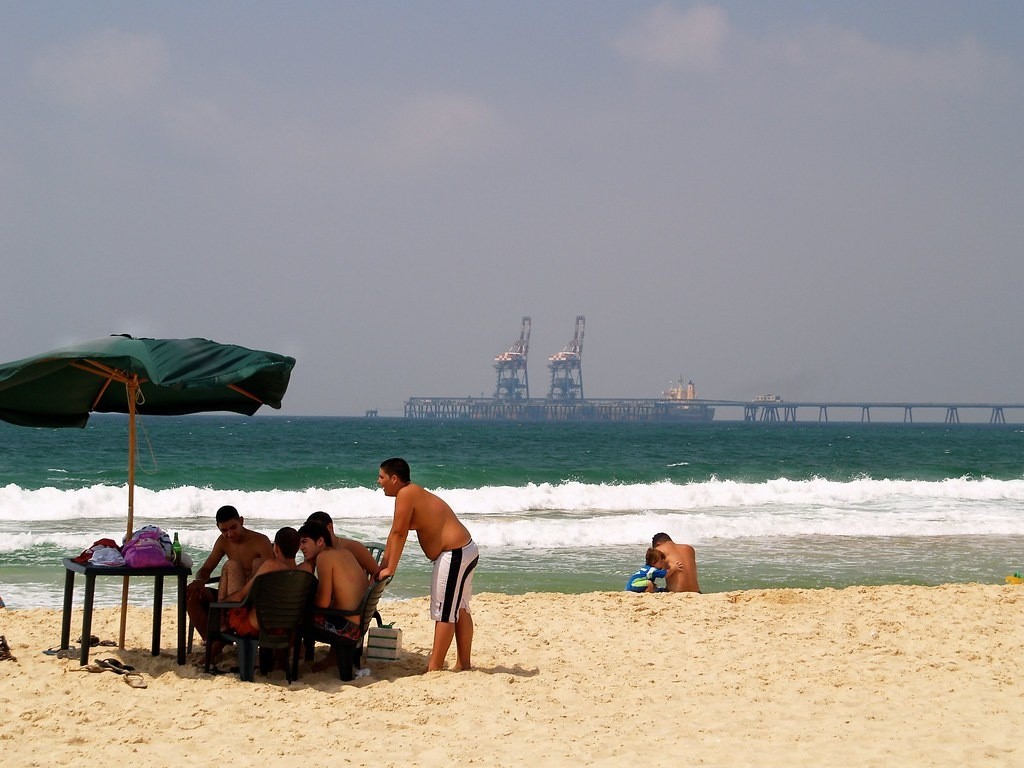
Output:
[184,505,379,666]
[652,532,703,594]
[626,548,683,593]
[369,457,479,673]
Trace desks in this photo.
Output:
[60,558,193,666]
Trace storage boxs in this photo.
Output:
[365,627,404,665]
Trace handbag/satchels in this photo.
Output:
[74,526,173,567]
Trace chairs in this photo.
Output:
[187,576,221,654]
[301,575,391,681]
[361,542,394,627]
[205,569,319,684]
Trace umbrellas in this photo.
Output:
[0,333,296,649]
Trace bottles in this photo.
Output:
[172,532,182,567]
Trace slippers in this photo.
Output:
[68,664,113,672]
[123,673,147,688]
[77,633,116,647]
[43,644,74,655]
[94,658,135,674]
[57,648,81,660]
